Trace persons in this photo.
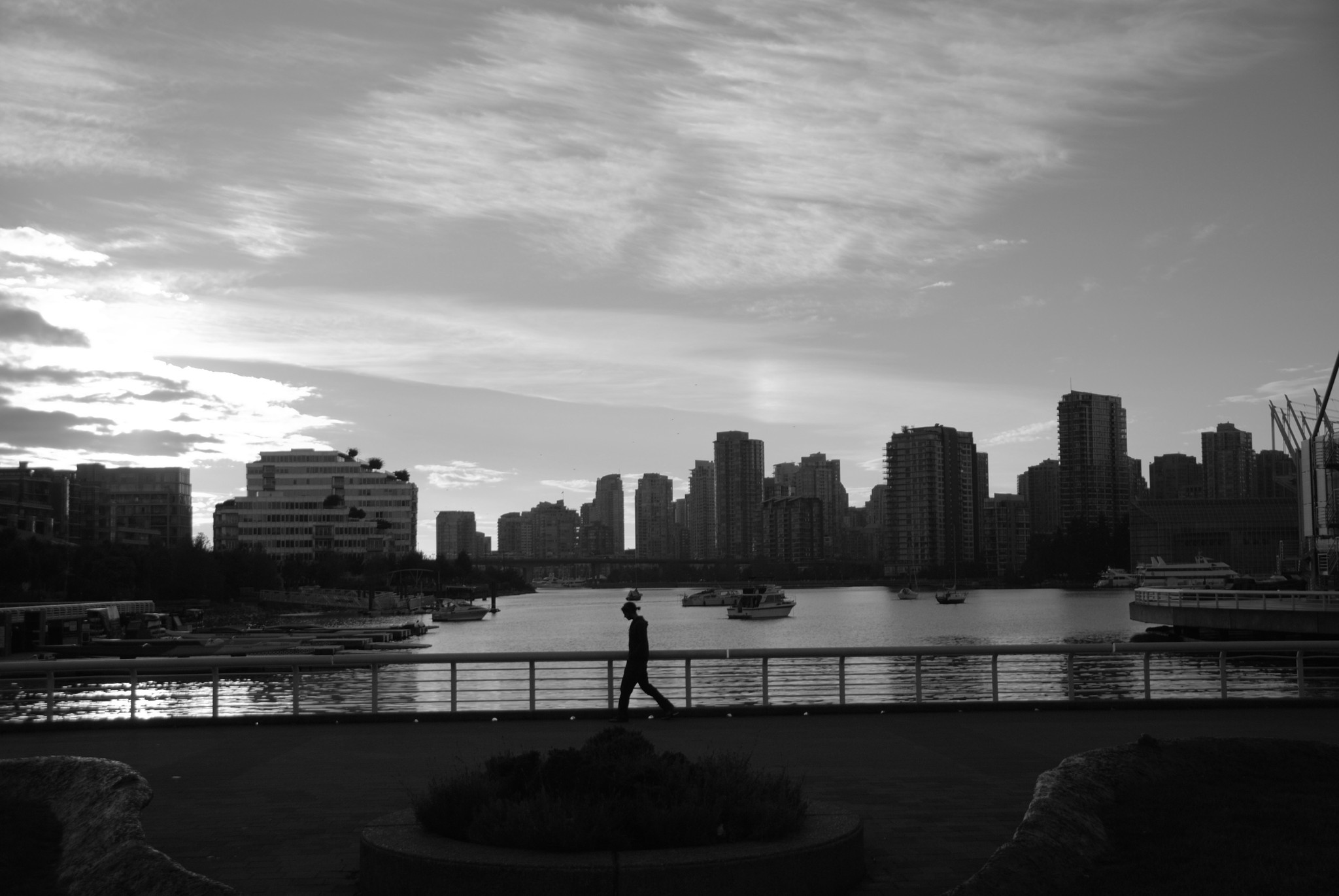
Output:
[608,602,679,723]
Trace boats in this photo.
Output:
[726,584,796,620]
[1095,566,1139,588]
[1136,555,1237,587]
[31,608,338,655]
[1257,575,1288,583]
[431,599,491,621]
[681,583,741,606]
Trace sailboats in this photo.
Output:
[936,523,968,604]
[897,540,919,600]
[532,564,592,588]
[626,552,643,601]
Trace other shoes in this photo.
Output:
[609,715,630,721]
[659,709,679,720]
